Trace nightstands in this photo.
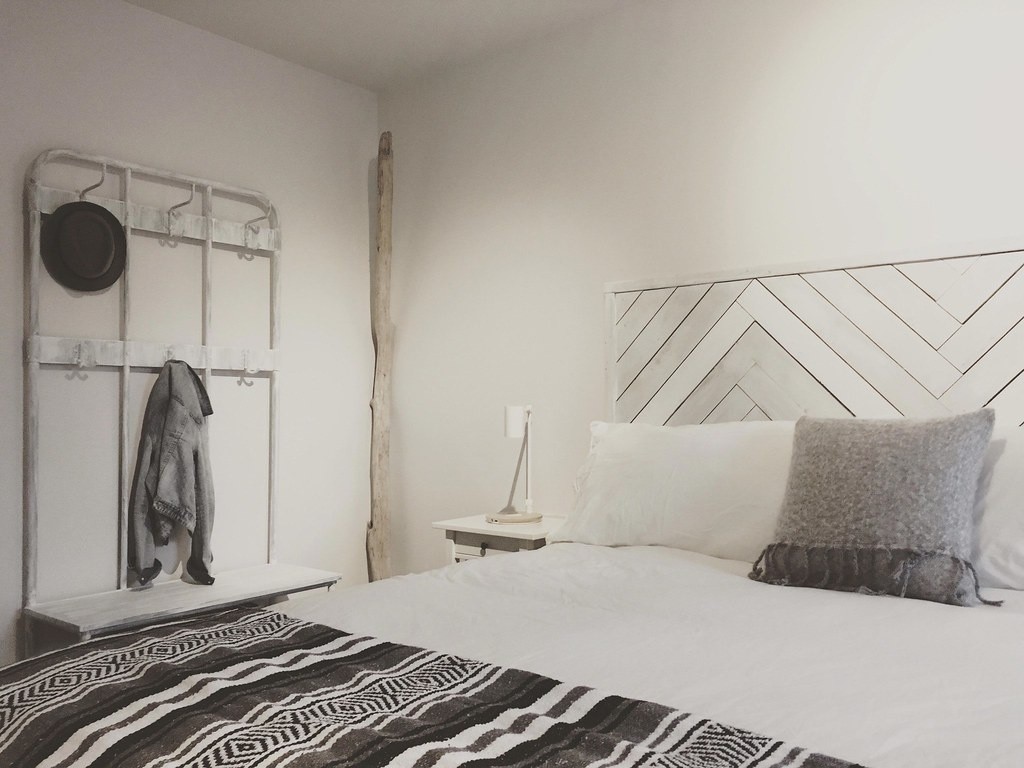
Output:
[432,513,555,564]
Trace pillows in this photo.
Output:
[746,407,1001,609]
[549,420,795,567]
[964,425,1024,590]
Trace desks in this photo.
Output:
[26,562,341,644]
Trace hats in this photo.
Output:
[40,200,127,291]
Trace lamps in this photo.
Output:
[485,405,542,523]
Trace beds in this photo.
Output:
[0,244,1024,768]
